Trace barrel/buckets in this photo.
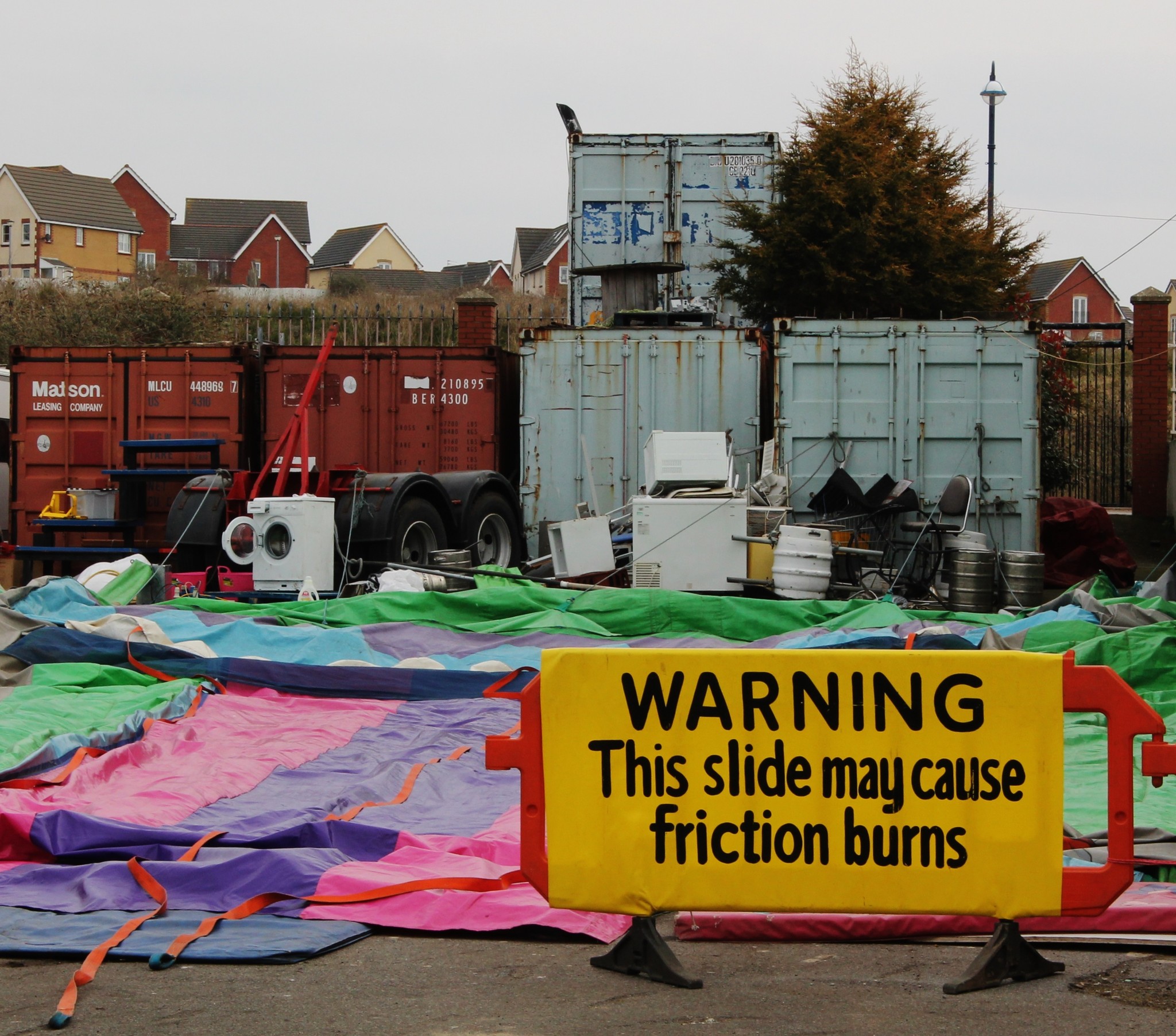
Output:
[170,566,212,597]
[998,550,1046,613]
[217,566,253,601]
[941,548,995,613]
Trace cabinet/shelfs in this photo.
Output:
[18,440,224,582]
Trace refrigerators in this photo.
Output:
[632,495,747,593]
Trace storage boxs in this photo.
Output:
[68,488,120,520]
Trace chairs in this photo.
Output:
[903,473,972,610]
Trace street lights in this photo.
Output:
[7,220,15,277]
[487,260,494,287]
[274,235,282,287]
[979,61,1008,248]
